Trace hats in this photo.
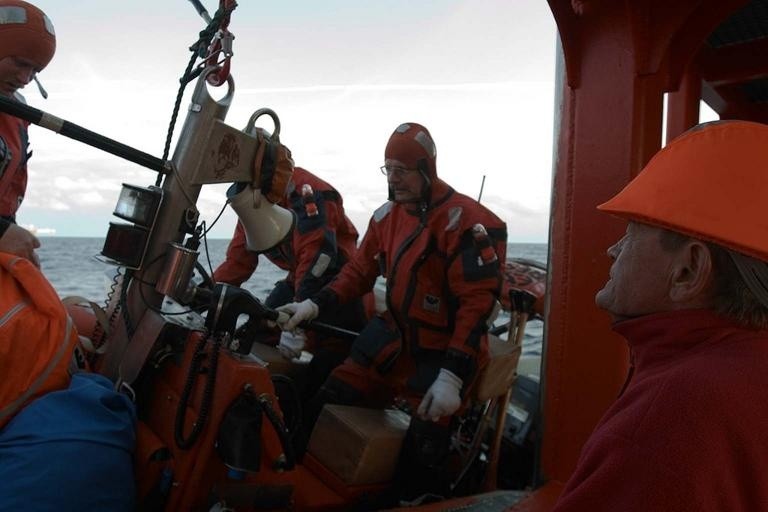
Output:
[385,123,436,169]
[0,0,56,66]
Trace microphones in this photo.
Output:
[34,75,48,100]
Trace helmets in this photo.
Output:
[596,119,768,262]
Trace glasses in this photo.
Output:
[381,166,418,174]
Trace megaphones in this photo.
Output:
[226,182,294,252]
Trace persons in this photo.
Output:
[278,123,506,487]
[1,1,55,272]
[198,125,378,462]
[556,120,768,512]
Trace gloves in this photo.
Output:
[417,368,463,422]
[268,298,320,330]
